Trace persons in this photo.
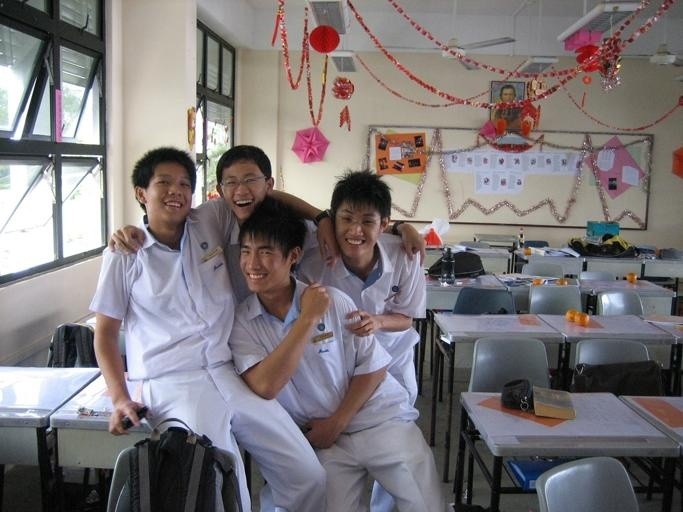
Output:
[226,198,449,512]
[86,146,340,512]
[105,146,428,303]
[492,85,522,127]
[295,168,429,407]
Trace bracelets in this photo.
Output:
[391,220,405,234]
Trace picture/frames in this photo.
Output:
[490,81,526,133]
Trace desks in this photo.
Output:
[49,370,246,510]
[537,313,678,393]
[0,365,100,511]
[415,274,508,395]
[618,394,681,510]
[434,312,566,484]
[451,392,681,510]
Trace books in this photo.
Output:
[532,385,576,420]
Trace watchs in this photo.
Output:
[313,208,331,227]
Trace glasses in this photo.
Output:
[220,175,265,189]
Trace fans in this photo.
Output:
[374,0,514,71]
[618,1,682,82]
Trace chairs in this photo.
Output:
[465,336,551,508]
[572,338,651,395]
[431,243,682,315]
[535,455,640,510]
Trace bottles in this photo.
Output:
[441,247,455,283]
[519,228,525,248]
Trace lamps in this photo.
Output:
[515,0,642,74]
[309,1,357,72]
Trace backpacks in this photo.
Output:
[114,418,243,511]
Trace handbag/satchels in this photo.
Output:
[502,378,532,410]
[428,251,485,279]
[574,360,665,396]
